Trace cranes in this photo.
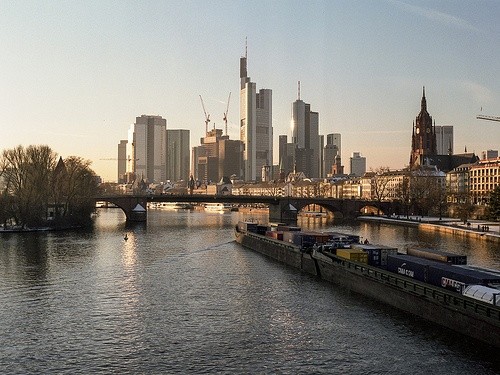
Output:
[223,92,231,135]
[199,94,210,136]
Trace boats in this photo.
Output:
[232,221,500,348]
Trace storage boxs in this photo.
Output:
[238,220,500,293]
